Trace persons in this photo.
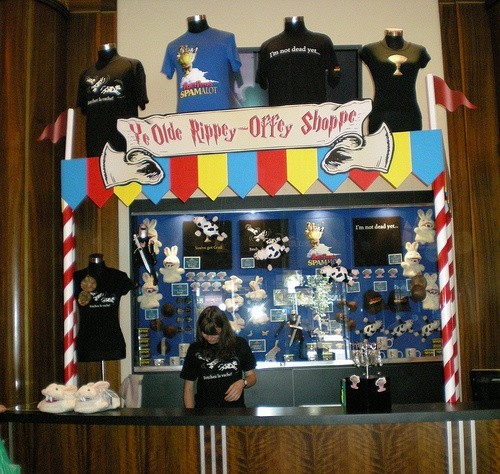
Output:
[254,16,340,107]
[73,44,148,158]
[73,253,133,362]
[358,29,431,134]
[159,16,241,113]
[180,306,257,409]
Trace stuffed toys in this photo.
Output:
[74,380,121,413]
[320,259,357,287]
[230,312,245,333]
[414,316,442,342]
[355,316,385,344]
[245,276,268,301]
[254,236,290,272]
[384,314,414,341]
[194,216,228,242]
[159,245,185,283]
[136,273,163,310]
[143,217,162,255]
[423,271,441,311]
[225,293,244,312]
[35,382,78,414]
[414,209,438,244]
[400,242,425,277]
[221,275,243,293]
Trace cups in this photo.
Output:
[386,349,403,359]
[169,356,185,366]
[179,343,190,357]
[153,358,165,366]
[376,337,393,350]
[377,351,385,360]
[405,348,421,358]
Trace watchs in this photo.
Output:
[243,379,248,388]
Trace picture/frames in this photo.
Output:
[322,44,363,104]
[232,44,271,108]
